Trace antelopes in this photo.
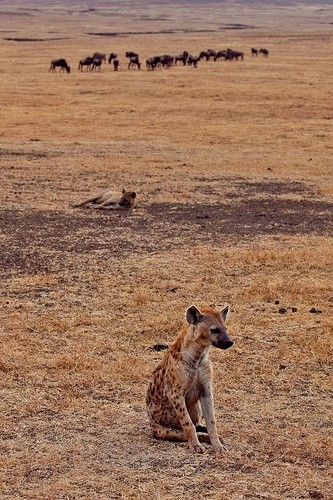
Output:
[78,47,269,72]
[48,59,70,73]
